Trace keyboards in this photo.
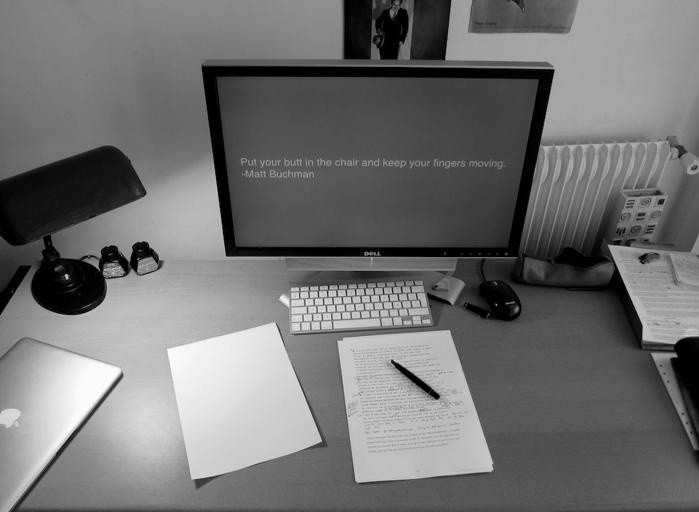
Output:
[289,276,433,335]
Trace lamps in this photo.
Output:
[0,144,146,316]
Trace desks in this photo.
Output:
[1,265,699,512]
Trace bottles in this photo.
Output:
[99,246,129,279]
[130,241,159,276]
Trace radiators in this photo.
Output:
[520,138,699,259]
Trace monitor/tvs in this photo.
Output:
[200,60,556,272]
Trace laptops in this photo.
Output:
[0,338,123,511]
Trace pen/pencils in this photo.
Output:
[390,358,441,402]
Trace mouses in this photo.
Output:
[478,279,521,321]
